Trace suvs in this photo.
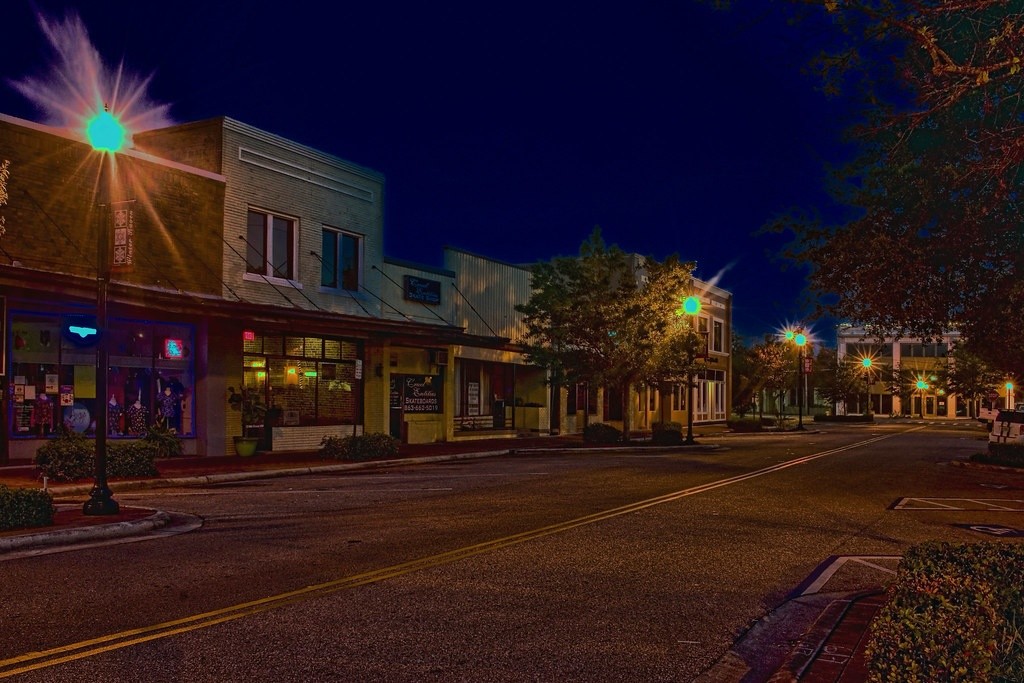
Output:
[987,409,1024,457]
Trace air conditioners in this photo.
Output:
[427,350,449,366]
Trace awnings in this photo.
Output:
[0,178,511,349]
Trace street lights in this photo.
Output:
[81,110,129,517]
[917,380,925,419]
[794,333,807,432]
[862,357,872,415]
[1006,383,1013,409]
[682,295,701,446]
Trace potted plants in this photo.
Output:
[228,384,267,456]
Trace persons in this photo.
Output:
[126,401,148,432]
[31,393,54,432]
[108,399,125,435]
[157,388,179,417]
